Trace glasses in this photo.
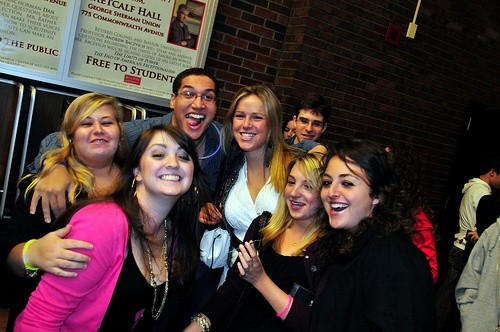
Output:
[296,117,324,129]
[176,91,217,104]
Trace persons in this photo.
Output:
[0,93,131,332]
[27,68,328,223]
[14,124,214,332]
[436,146,500,332]
[198,85,441,332]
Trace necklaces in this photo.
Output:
[143,218,169,320]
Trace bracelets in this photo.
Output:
[275,294,293,317]
[22,238,38,277]
[191,314,210,332]
